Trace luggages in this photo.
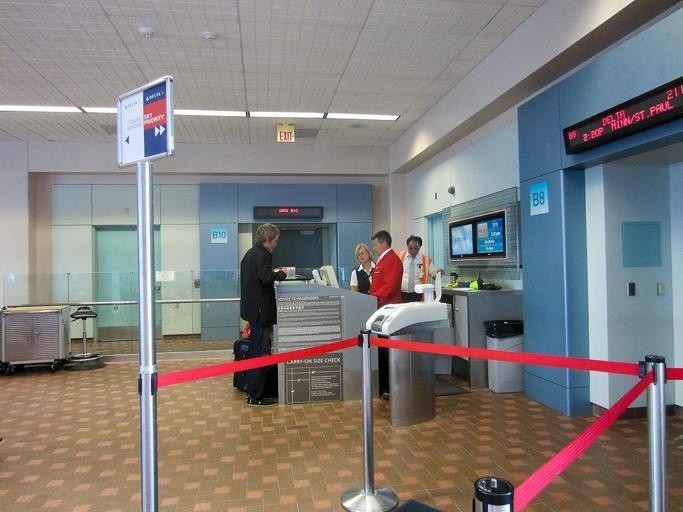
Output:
[233,340,249,392]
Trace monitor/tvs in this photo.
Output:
[320,266,339,288]
[449,211,506,259]
[310,269,321,284]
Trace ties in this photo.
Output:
[408,257,416,293]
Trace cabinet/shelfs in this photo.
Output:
[452,291,523,388]
[1,303,71,377]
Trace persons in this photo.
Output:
[240,224,289,407]
[349,230,446,309]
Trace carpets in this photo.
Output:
[435,375,471,396]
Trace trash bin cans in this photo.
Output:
[483,320,524,393]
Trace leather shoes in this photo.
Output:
[248,396,278,406]
